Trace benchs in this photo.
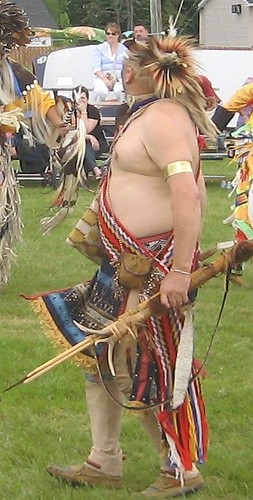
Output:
[98,117,234,182]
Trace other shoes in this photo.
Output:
[230,263,243,276]
[134,472,204,500]
[46,464,124,490]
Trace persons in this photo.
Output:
[57,85,109,181]
[198,77,253,285]
[124,24,148,51]
[0,33,72,287]
[46,33,206,499]
[88,22,130,109]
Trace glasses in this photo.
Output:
[105,32,118,35]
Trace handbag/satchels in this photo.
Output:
[117,251,153,291]
[65,192,109,267]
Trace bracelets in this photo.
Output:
[170,269,190,275]
[80,112,87,115]
